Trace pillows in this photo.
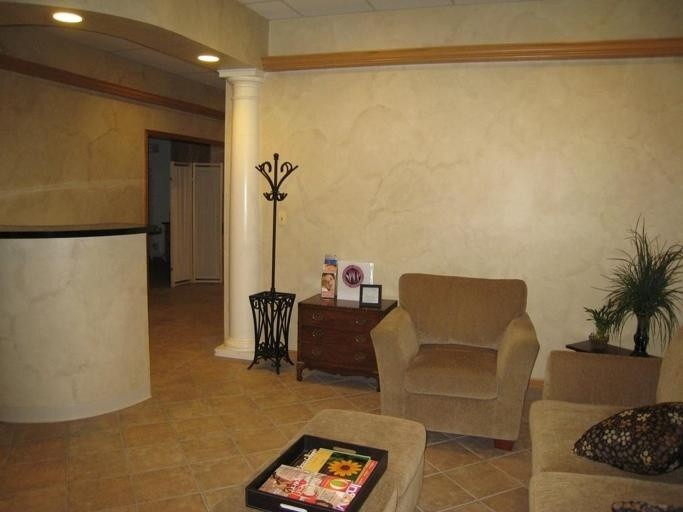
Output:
[572,401,683,474]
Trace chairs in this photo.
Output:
[370,272,540,450]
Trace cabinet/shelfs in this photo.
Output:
[295,293,397,393]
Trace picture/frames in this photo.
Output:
[359,284,382,309]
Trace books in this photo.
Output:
[258,447,378,511]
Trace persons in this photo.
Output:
[321,274,335,291]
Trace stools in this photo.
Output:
[210,409,429,512]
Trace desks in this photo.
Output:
[566,336,651,356]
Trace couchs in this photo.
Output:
[527,324,683,512]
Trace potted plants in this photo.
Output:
[600,212,683,357]
[583,301,619,346]
